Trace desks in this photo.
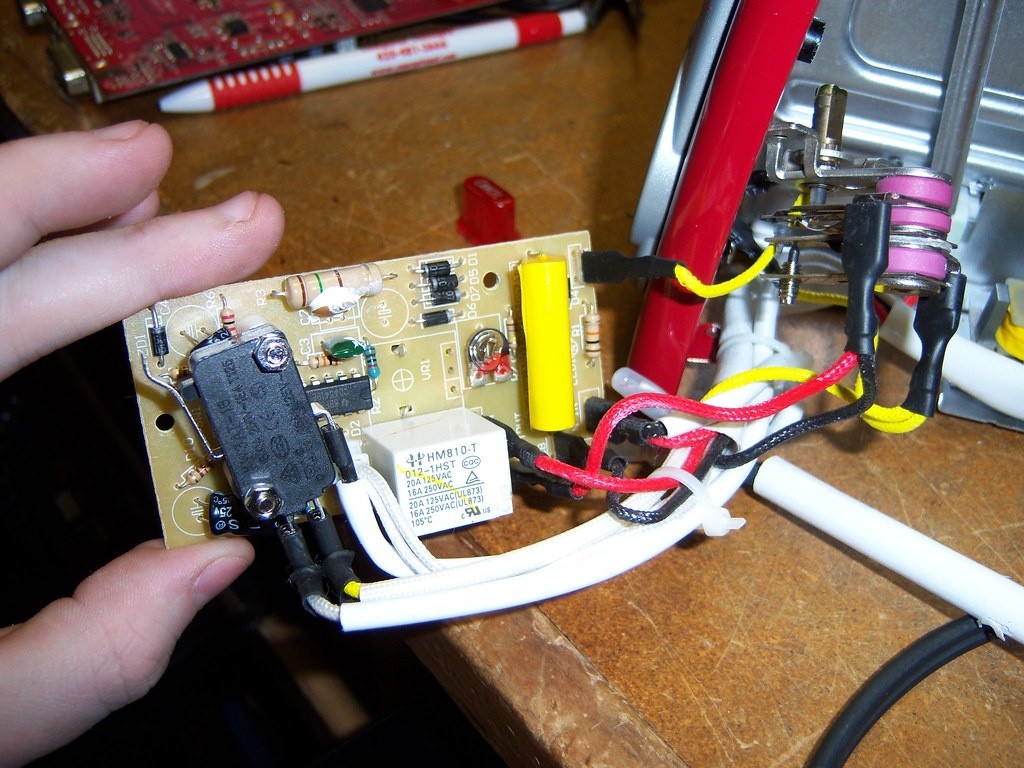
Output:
[0,1,1024,768]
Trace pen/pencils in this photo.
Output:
[159,8,586,114]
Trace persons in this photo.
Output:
[0,119,286,768]
